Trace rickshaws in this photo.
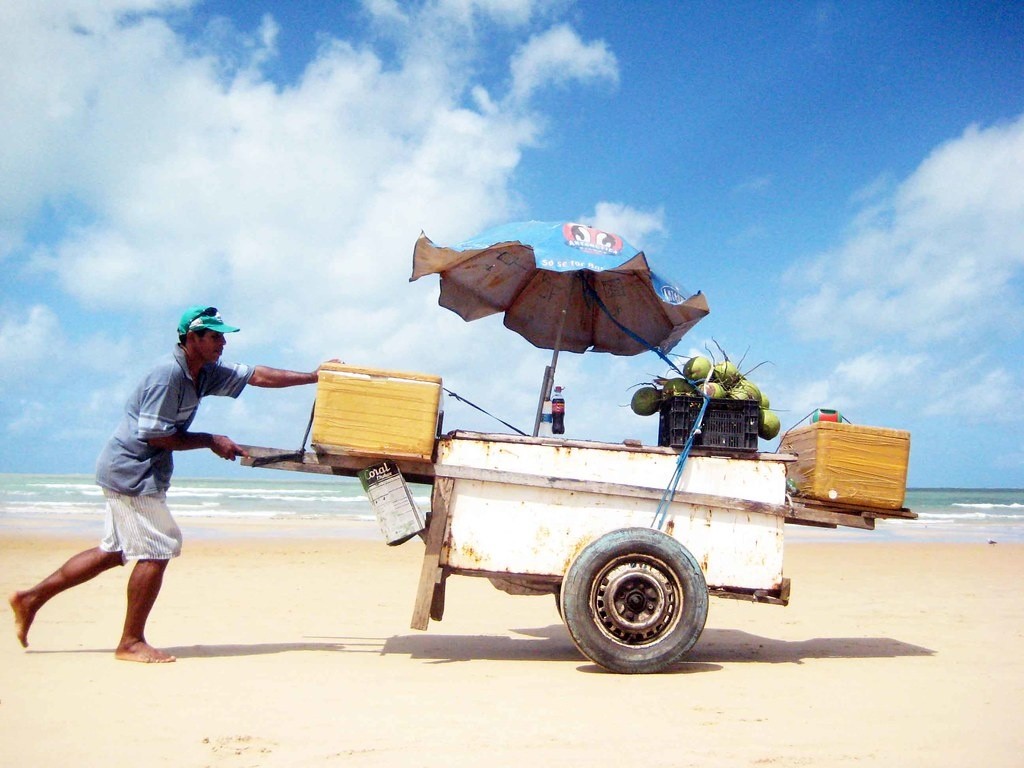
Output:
[234,430,918,675]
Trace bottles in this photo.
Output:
[539,397,553,437]
[552,386,565,435]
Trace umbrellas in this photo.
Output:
[408,219,709,400]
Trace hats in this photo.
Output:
[177,305,240,336]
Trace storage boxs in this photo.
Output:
[312,362,445,460]
[657,395,761,448]
[780,420,912,508]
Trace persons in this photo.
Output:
[7,306,346,664]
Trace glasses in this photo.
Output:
[186,307,217,328]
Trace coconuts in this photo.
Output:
[631,355,783,441]
